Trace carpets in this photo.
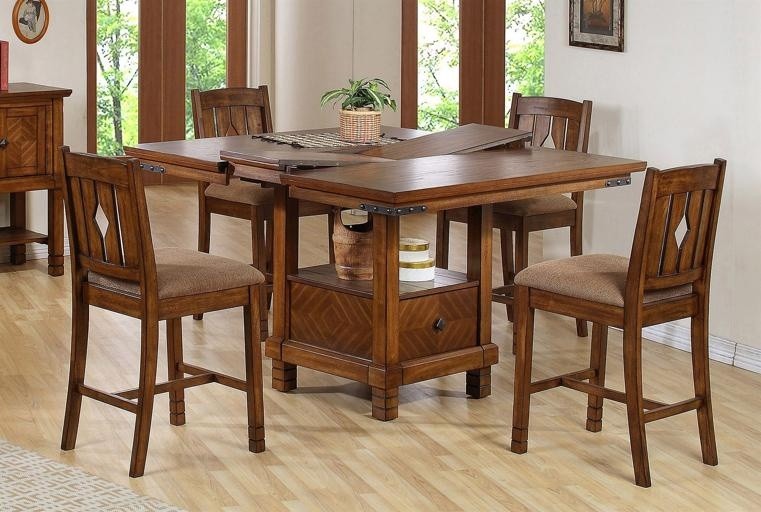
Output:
[0,440,186,512]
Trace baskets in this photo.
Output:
[338,110,381,143]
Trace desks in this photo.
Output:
[121,123,648,423]
[0,82,74,277]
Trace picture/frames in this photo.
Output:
[13,0,50,44]
[567,0,626,53]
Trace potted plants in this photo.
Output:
[321,75,398,142]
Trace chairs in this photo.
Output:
[507,156,728,489]
[434,91,593,354]
[55,146,267,477]
[190,85,337,340]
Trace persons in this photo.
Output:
[23,0,38,32]
